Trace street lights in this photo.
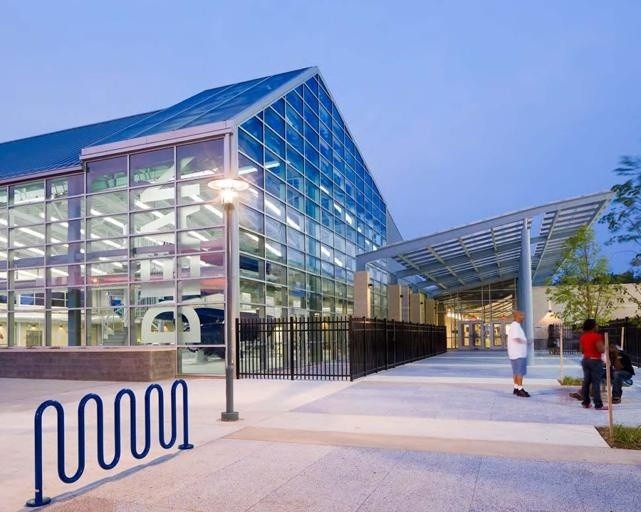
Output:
[208,177,249,421]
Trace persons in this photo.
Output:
[507,311,529,397]
[547,319,635,409]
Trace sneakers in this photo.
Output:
[514,388,530,397]
[583,403,592,408]
[568,392,583,401]
[595,405,609,410]
[612,397,621,404]
